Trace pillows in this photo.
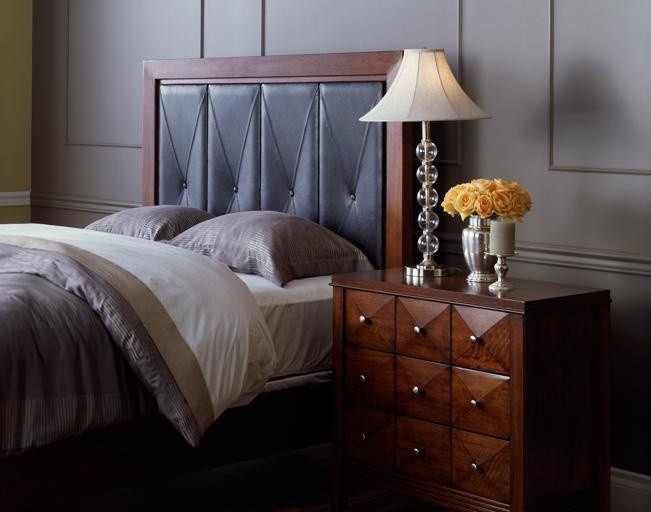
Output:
[159,210,375,289]
[84,205,217,247]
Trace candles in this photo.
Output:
[489,217,515,255]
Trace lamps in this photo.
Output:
[357,47,491,277]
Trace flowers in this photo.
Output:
[440,177,532,224]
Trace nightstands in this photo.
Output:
[329,267,614,512]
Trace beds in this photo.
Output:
[0,50,407,512]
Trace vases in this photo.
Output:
[462,213,498,283]
